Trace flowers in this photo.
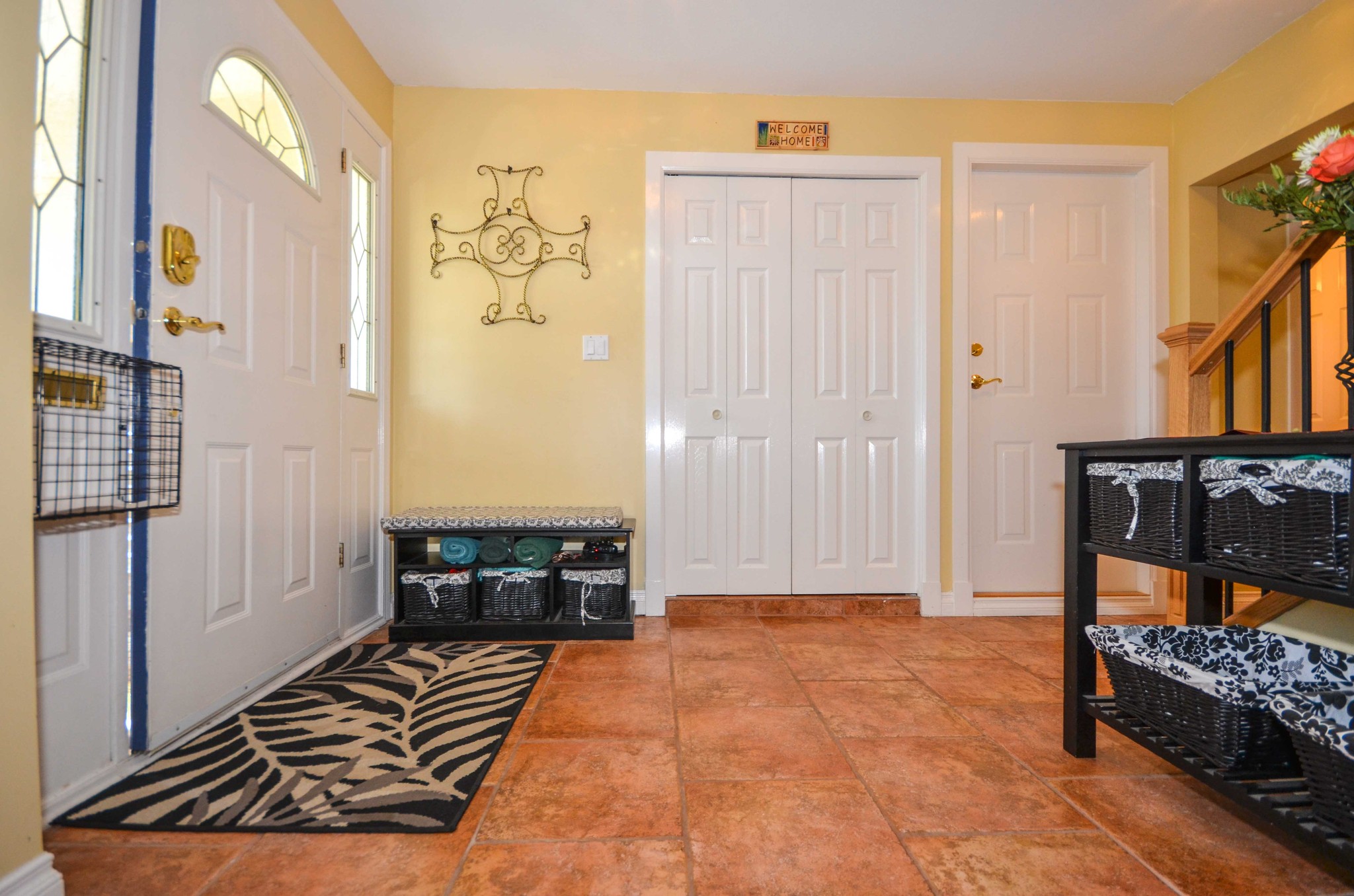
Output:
[1219,124,1354,249]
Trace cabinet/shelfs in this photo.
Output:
[1053,429,1354,886]
[379,505,636,640]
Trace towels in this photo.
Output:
[478,536,511,564]
[513,535,564,569]
[439,536,481,564]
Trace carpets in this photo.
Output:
[46,640,557,835]
